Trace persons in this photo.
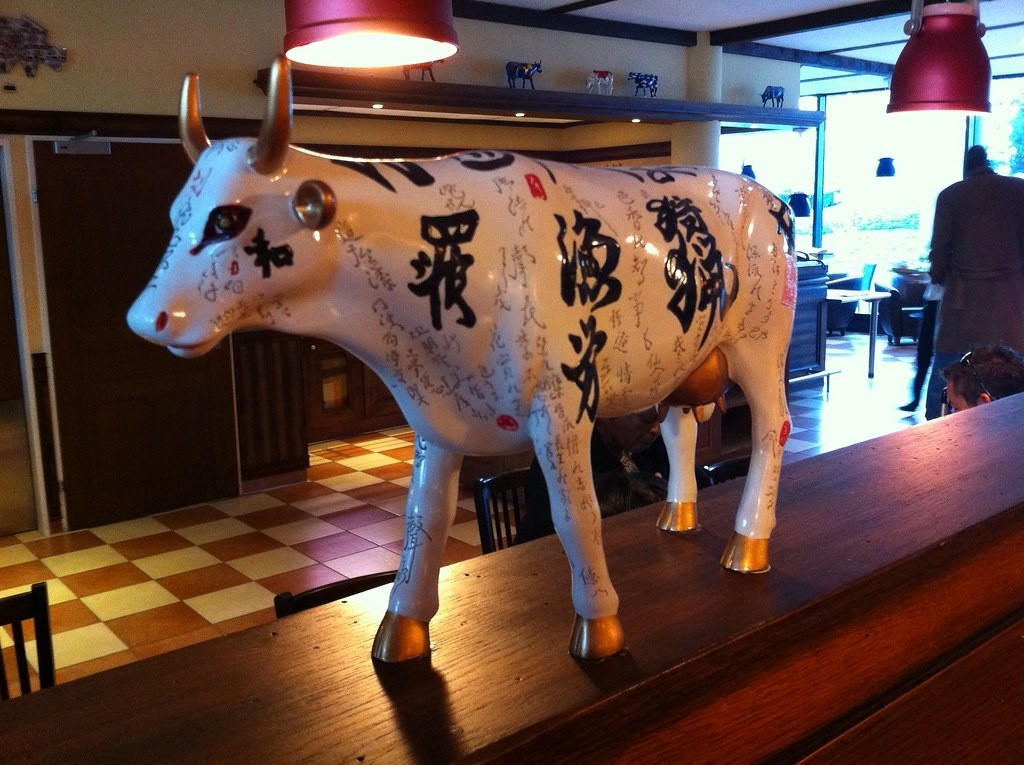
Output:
[898,144,1024,423]
[514,397,714,546]
[940,344,1024,412]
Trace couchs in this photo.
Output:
[874,283,924,346]
[825,276,863,336]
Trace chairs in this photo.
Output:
[475,466,535,556]
[0,582,56,704]
[857,264,877,314]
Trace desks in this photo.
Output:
[827,288,891,378]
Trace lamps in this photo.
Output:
[875,157,897,177]
[741,164,756,179]
[786,193,812,217]
[884,1,993,117]
[282,0,461,67]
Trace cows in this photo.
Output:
[125,51,799,657]
